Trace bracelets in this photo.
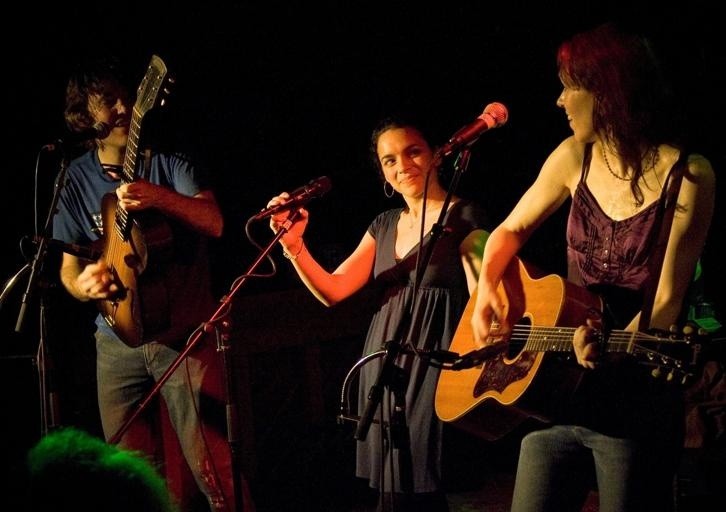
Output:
[282,238,306,260]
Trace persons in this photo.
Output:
[51,70,256,512]
[474,27,715,512]
[266,121,484,512]
[22,425,176,512]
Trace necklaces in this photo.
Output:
[601,150,660,181]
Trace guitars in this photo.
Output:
[434,255,705,421]
[92,54,177,347]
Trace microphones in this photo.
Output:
[25,233,101,262]
[255,176,331,221]
[54,121,111,152]
[442,102,508,154]
